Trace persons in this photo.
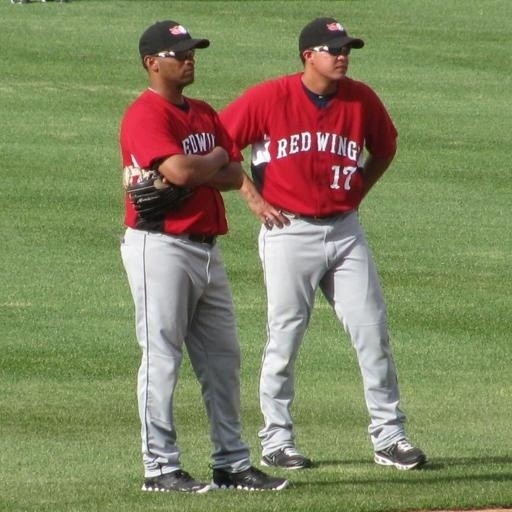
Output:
[117,18,287,494]
[219,18,421,471]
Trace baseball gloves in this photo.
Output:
[126,169,197,223]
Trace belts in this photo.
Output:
[279,209,354,221]
[188,232,218,246]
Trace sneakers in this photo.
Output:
[373,437,428,471]
[259,443,311,471]
[139,468,211,495]
[207,462,290,492]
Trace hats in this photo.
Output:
[138,19,210,54]
[298,16,364,50]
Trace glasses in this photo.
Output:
[311,46,351,55]
[152,49,195,61]
